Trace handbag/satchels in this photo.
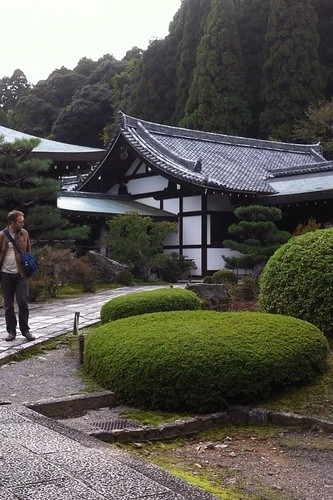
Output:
[21,252,40,277]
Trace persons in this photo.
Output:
[0,210,36,341]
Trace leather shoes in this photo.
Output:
[22,330,36,340]
[6,331,16,341]
